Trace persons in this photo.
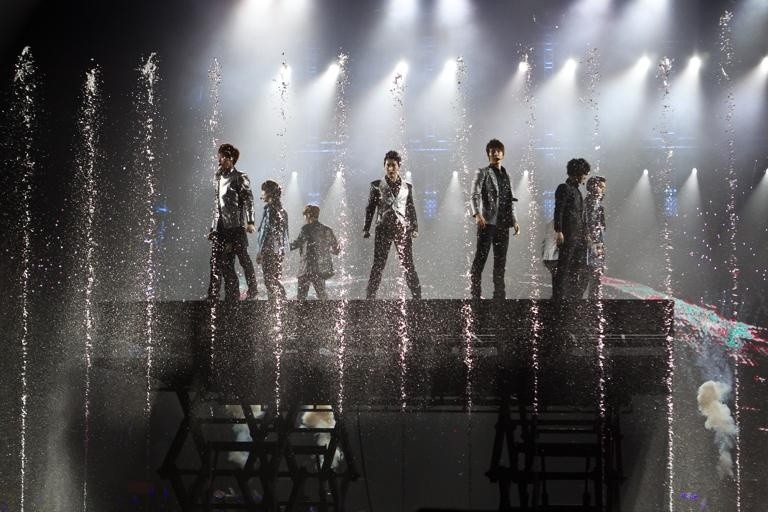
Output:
[541,157,607,300]
[363,150,422,300]
[205,143,340,300]
[470,138,520,300]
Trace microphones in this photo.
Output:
[496,157,504,160]
[603,194,605,196]
[219,158,231,166]
[390,168,400,175]
[582,181,585,185]
[260,197,264,200]
